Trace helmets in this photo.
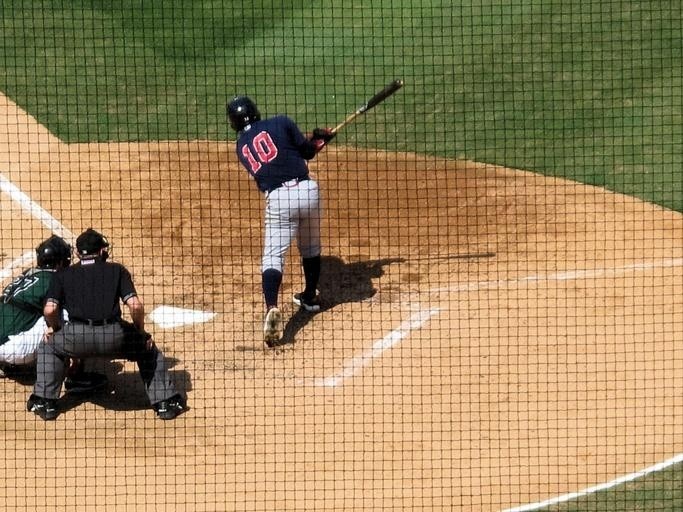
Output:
[37,236,71,269]
[227,97,261,132]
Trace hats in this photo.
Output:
[76,229,109,255]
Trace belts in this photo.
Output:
[268,175,310,194]
[0,336,10,345]
[72,318,117,325]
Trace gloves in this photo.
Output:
[313,128,335,151]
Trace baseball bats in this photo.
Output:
[331,79,405,135]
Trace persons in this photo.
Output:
[225,95,333,349]
[28,226,188,424]
[0,234,109,397]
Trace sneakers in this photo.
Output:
[157,393,186,419]
[65,372,108,390]
[264,306,281,344]
[27,395,56,420]
[292,293,320,311]
